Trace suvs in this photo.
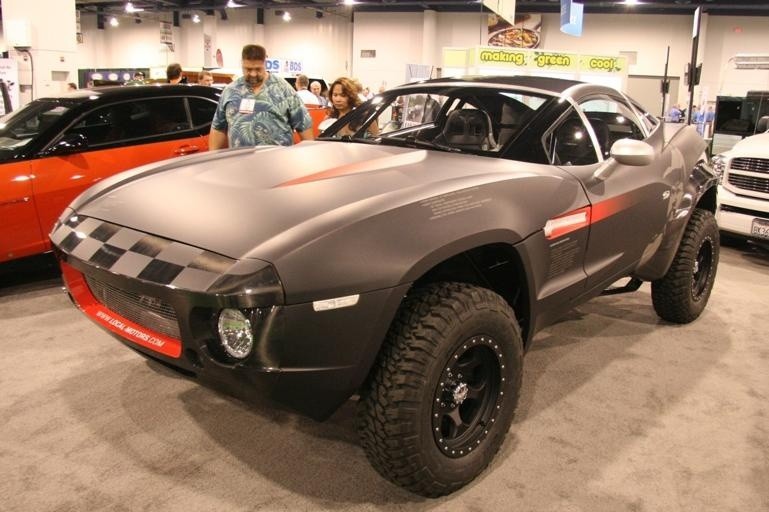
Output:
[48,74,720,497]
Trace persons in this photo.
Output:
[210,44,314,152]
[87,81,94,88]
[295,75,379,139]
[69,83,77,90]
[198,70,214,86]
[670,103,716,138]
[167,63,183,83]
[134,71,145,81]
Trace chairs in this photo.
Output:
[427,108,610,166]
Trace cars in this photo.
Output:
[0,83,333,267]
[711,113,769,246]
